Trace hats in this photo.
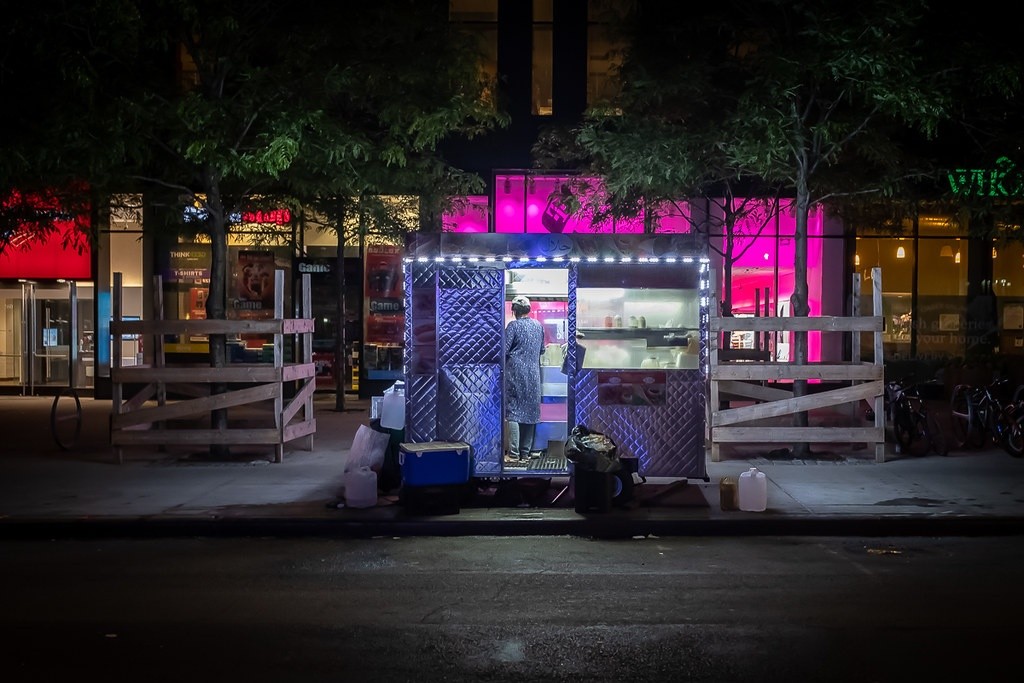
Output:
[511,296,530,306]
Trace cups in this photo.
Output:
[542,362,548,366]
[659,361,667,368]
[643,377,655,384]
[623,391,632,403]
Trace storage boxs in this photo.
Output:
[399,441,470,485]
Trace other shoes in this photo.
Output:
[504,455,520,463]
[519,457,531,464]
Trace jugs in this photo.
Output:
[549,344,560,365]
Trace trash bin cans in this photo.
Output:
[370,418,405,488]
[575,461,613,513]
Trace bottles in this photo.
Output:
[604,316,612,326]
[614,316,622,325]
[628,315,639,327]
[639,316,646,328]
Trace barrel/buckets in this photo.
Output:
[344,466,379,508]
[380,379,406,431]
[739,468,768,512]
[721,476,737,510]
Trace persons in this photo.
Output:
[505,295,546,462]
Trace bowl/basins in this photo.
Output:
[615,370,625,383]
[647,386,663,397]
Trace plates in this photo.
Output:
[609,377,621,383]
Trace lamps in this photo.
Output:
[855,239,859,266]
[528,177,535,193]
[940,240,960,263]
[994,245,998,258]
[789,203,816,218]
[897,236,906,258]
[554,178,560,192]
[504,177,511,193]
